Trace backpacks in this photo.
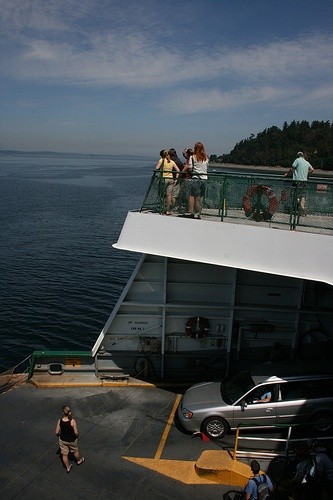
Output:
[252,474,272,500]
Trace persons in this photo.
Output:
[284,152,315,216]
[155,142,208,219]
[244,460,273,500]
[253,387,271,403]
[55,405,84,473]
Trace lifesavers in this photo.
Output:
[244,185,278,219]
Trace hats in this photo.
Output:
[251,460,260,472]
[296,152,303,157]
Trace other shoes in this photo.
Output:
[184,211,200,220]
[77,457,85,465]
[162,209,173,215]
[67,463,73,473]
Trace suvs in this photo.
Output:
[177,364,317,440]
